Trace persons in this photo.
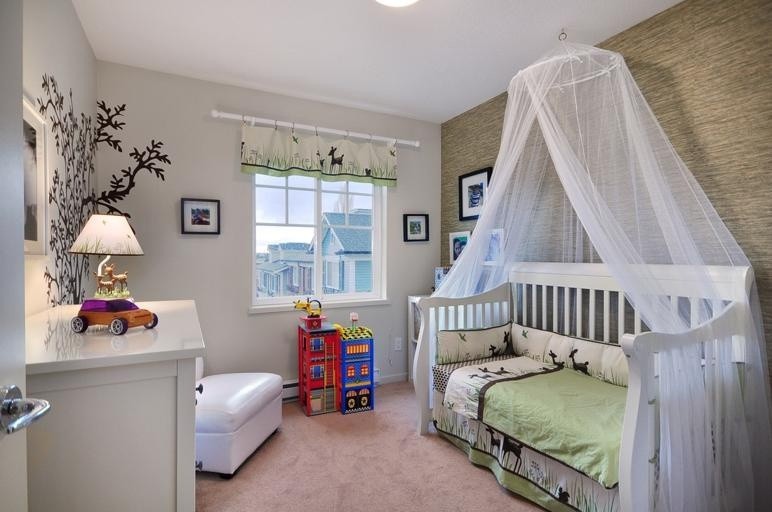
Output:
[469,187,481,209]
[454,238,460,260]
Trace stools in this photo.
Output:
[195,372,285,478]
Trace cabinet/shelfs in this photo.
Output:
[26,297,205,511]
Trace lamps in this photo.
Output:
[68,214,145,303]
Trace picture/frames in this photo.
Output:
[458,167,493,221]
[23,93,49,256]
[181,198,220,235]
[449,228,505,267]
[404,214,430,242]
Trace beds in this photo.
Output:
[414,263,756,512]
